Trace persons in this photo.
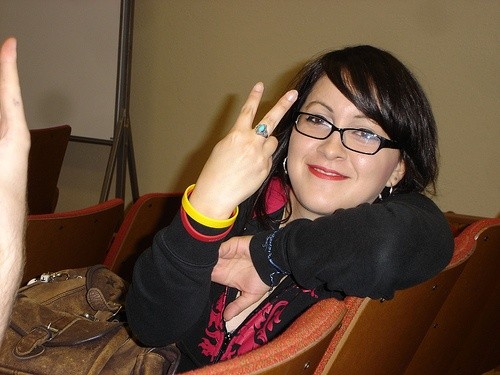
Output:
[123,46,453,373]
[0,37,31,345]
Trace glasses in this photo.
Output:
[294,112,400,155]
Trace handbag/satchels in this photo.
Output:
[0,265,179,375]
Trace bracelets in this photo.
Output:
[181,183,239,228]
[180,204,235,242]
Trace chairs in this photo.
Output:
[20,195,128,290]
[106,193,500,375]
[27,124,73,216]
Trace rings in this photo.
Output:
[256,124,268,138]
